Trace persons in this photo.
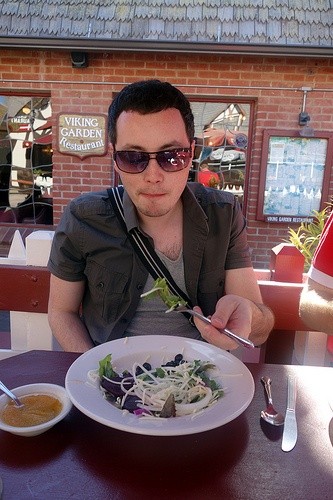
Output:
[299,208,333,336]
[47,78,275,352]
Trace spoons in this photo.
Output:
[259,375,285,426]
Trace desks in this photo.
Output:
[0,350,333,500]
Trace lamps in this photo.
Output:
[70,52,87,69]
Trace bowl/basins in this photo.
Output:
[64,335,255,436]
[0,383,73,437]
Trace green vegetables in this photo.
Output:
[140,278,189,314]
[98,353,224,398]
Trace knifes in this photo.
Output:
[280,373,298,452]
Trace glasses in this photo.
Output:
[111,147,192,175]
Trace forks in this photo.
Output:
[158,286,255,350]
